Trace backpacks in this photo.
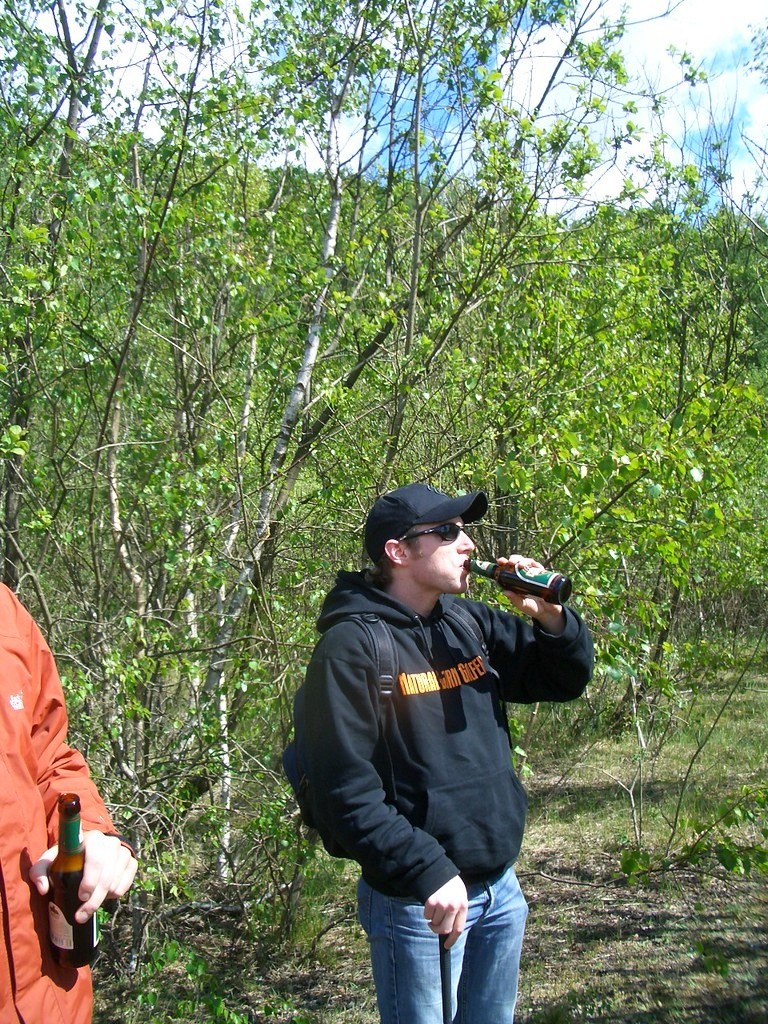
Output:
[282,602,490,859]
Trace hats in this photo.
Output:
[365,483,488,564]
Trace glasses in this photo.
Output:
[382,522,466,558]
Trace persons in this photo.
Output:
[0,581,139,1024]
[302,484,595,1024]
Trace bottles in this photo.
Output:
[464,558,572,606]
[46,793,100,968]
